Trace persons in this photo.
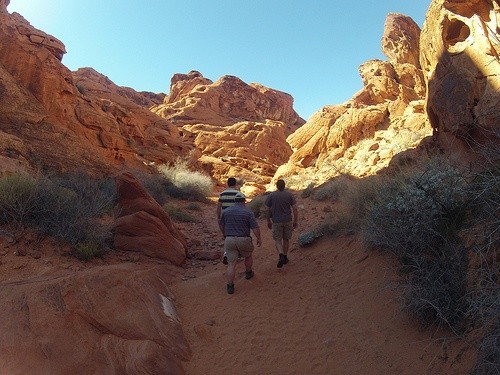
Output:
[216,177,240,264]
[266,180,298,268]
[220,193,262,295]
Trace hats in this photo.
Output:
[235,193,246,199]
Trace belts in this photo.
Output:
[226,234,246,238]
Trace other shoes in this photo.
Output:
[277,254,288,267]
[245,271,254,280]
[222,252,227,264]
[226,283,234,294]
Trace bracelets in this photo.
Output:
[257,240,261,241]
